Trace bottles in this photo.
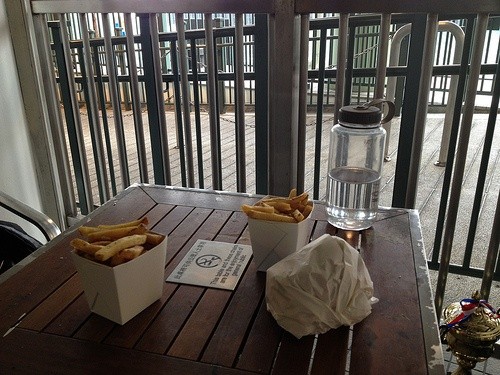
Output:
[325,99,395,231]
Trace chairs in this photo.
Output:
[1,190,58,274]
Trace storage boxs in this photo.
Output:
[247,196,314,272]
[73,230,167,326]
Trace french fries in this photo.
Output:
[70,216,163,268]
[242,190,314,223]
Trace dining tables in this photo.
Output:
[0,182,445,375]
